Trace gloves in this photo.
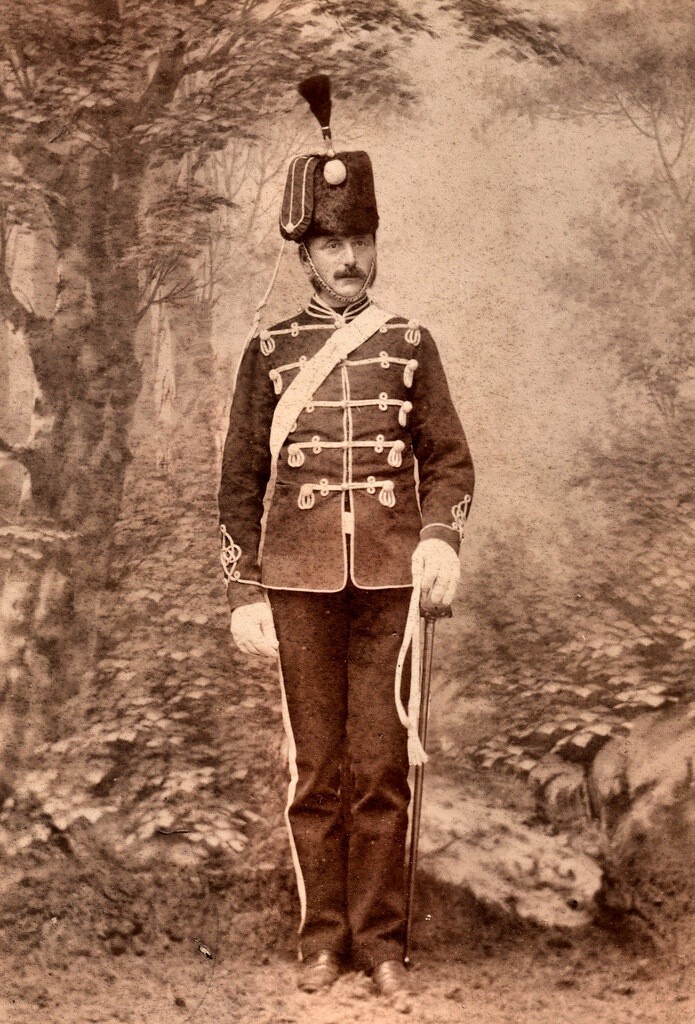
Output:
[410,538,461,607]
[230,602,280,665]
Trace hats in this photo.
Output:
[278,151,380,244]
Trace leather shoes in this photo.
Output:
[298,949,343,992]
[371,958,414,995]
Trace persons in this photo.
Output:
[217,150,475,1014]
